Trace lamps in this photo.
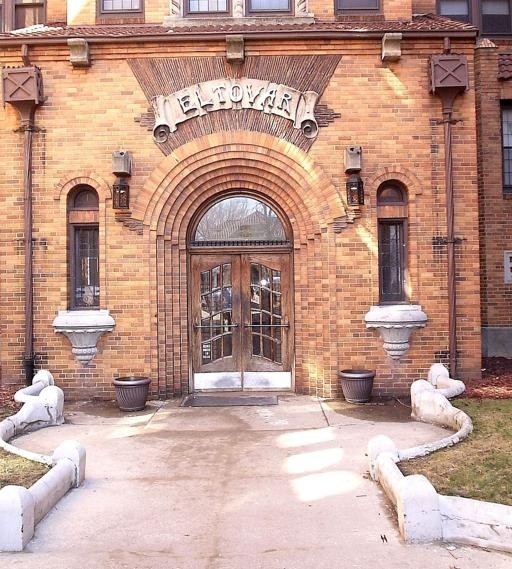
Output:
[346,173,364,206]
[112,175,129,210]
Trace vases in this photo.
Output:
[114,374,151,412]
[339,368,376,404]
